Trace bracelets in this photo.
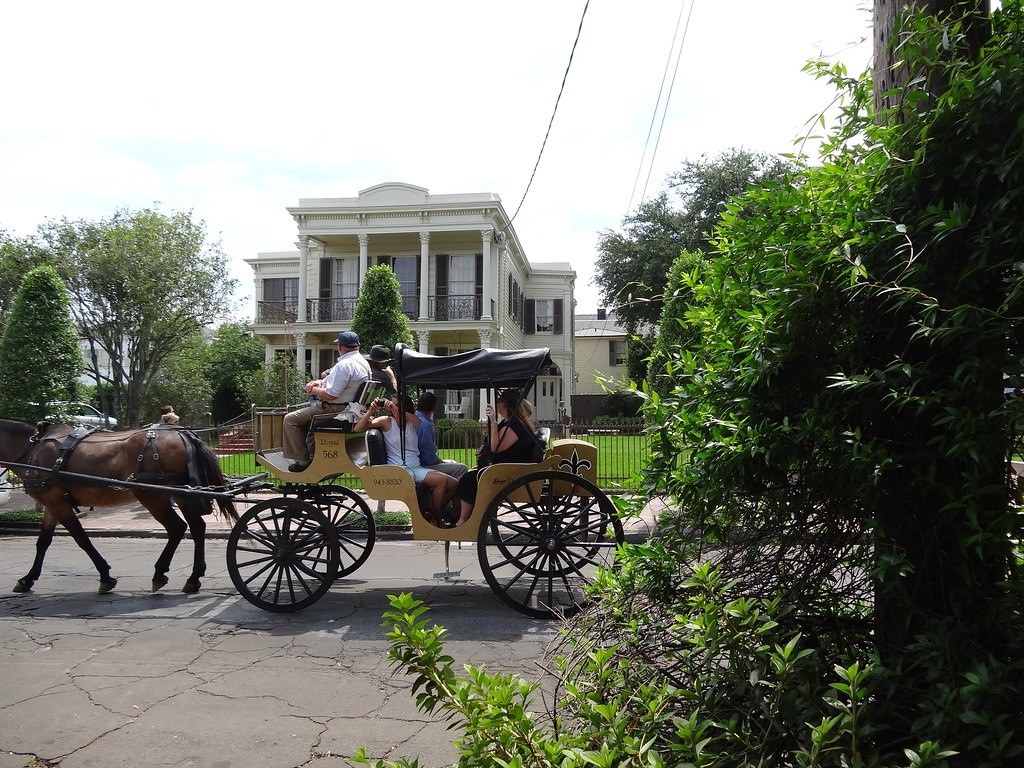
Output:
[490,421,498,425]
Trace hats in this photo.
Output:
[364,344,392,362]
[333,332,359,347]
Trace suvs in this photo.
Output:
[28,401,118,429]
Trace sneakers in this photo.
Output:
[288,461,312,472]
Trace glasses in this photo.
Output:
[497,398,501,402]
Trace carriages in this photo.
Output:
[0,343,625,620]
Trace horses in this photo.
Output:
[0,415,257,595]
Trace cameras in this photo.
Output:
[376,399,385,407]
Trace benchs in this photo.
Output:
[365,429,432,497]
[306,380,390,434]
[477,427,551,483]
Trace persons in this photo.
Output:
[450,390,547,530]
[322,344,397,395]
[413,392,468,508]
[353,393,458,530]
[283,331,372,472]
[162,406,179,424]
